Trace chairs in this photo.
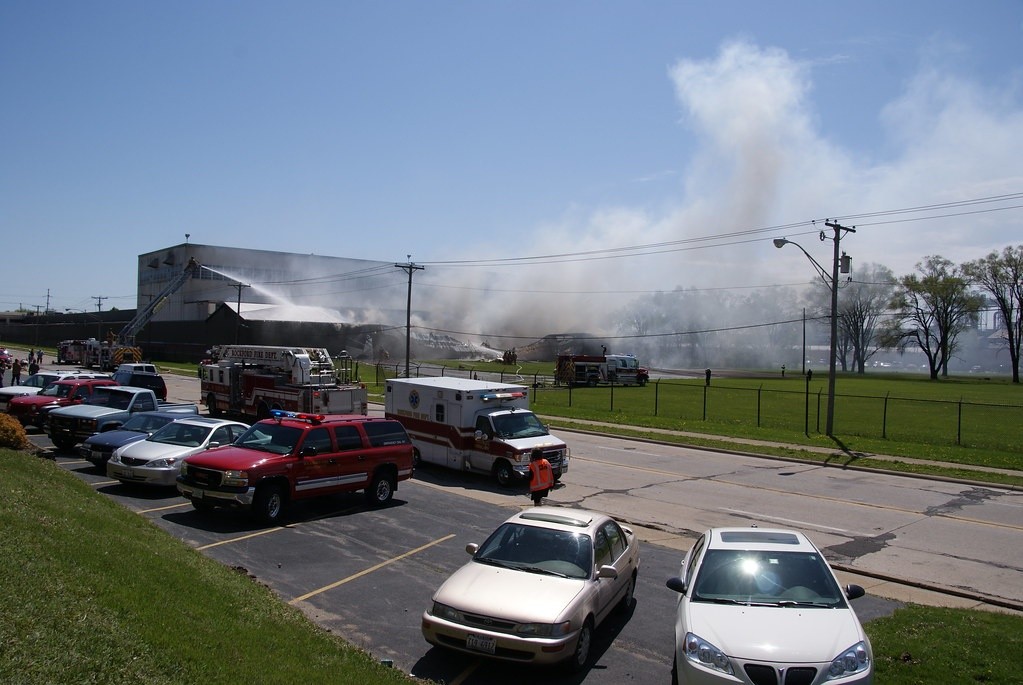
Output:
[191,429,201,441]
[505,534,550,563]
[151,421,165,432]
[212,430,227,444]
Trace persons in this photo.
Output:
[27,349,44,364]
[107,328,117,347]
[189,256,199,271]
[28,359,40,386]
[781,364,785,377]
[504,351,516,365]
[11,359,21,386]
[0,359,5,388]
[705,368,711,385]
[522,449,554,506]
[601,345,606,357]
[807,369,812,380]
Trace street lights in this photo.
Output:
[774,239,838,436]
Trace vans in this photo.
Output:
[118,363,157,373]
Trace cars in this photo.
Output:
[420,506,640,676]
[666,526,874,684]
[80,412,202,467]
[106,417,272,487]
[0,349,12,369]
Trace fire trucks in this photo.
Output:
[81,258,201,372]
[553,353,649,388]
[197,345,367,426]
[57,339,87,365]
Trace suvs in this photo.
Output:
[7,379,120,433]
[111,370,167,401]
[0,370,113,410]
[175,409,416,525]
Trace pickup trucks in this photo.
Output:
[42,386,198,450]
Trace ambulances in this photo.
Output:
[385,375,571,487]
